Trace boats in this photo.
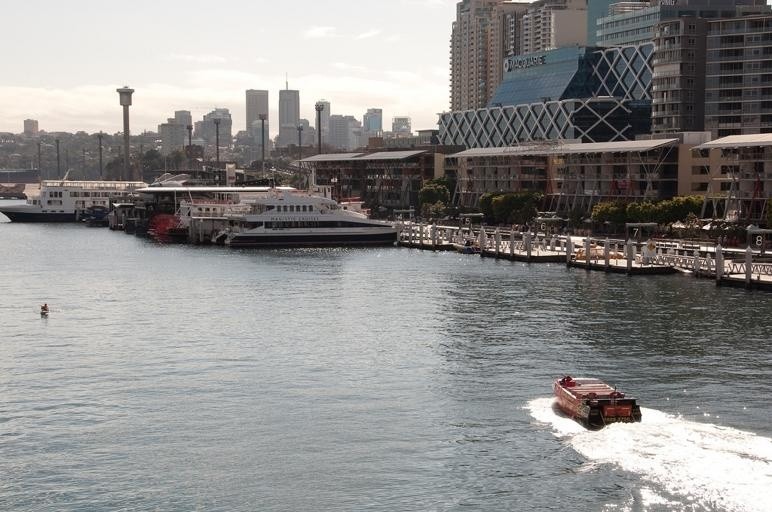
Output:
[40,305,49,318]
[551,372,642,430]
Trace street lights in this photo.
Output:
[37,103,331,190]
[116,85,135,178]
